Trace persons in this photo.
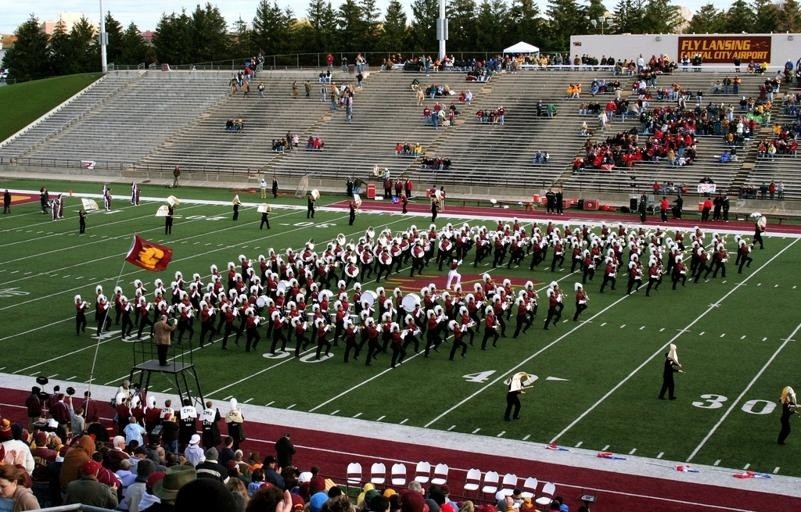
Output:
[776,386,800,446]
[2,190,10,213]
[373,49,801,182]
[658,343,682,401]
[40,176,786,372]
[172,164,181,187]
[502,373,525,420]
[225,49,367,152]
[0,378,570,512]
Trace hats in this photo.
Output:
[80,459,103,476]
[152,465,197,501]
[297,470,314,485]
[204,445,220,460]
[189,433,201,443]
[148,472,168,491]
[35,433,48,447]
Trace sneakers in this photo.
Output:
[545,211,566,216]
[712,219,729,222]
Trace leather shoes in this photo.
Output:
[505,415,520,421]
[75,321,261,351]
[269,348,421,368]
[658,396,677,400]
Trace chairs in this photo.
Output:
[344,461,555,512]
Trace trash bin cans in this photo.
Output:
[367,184,376,199]
